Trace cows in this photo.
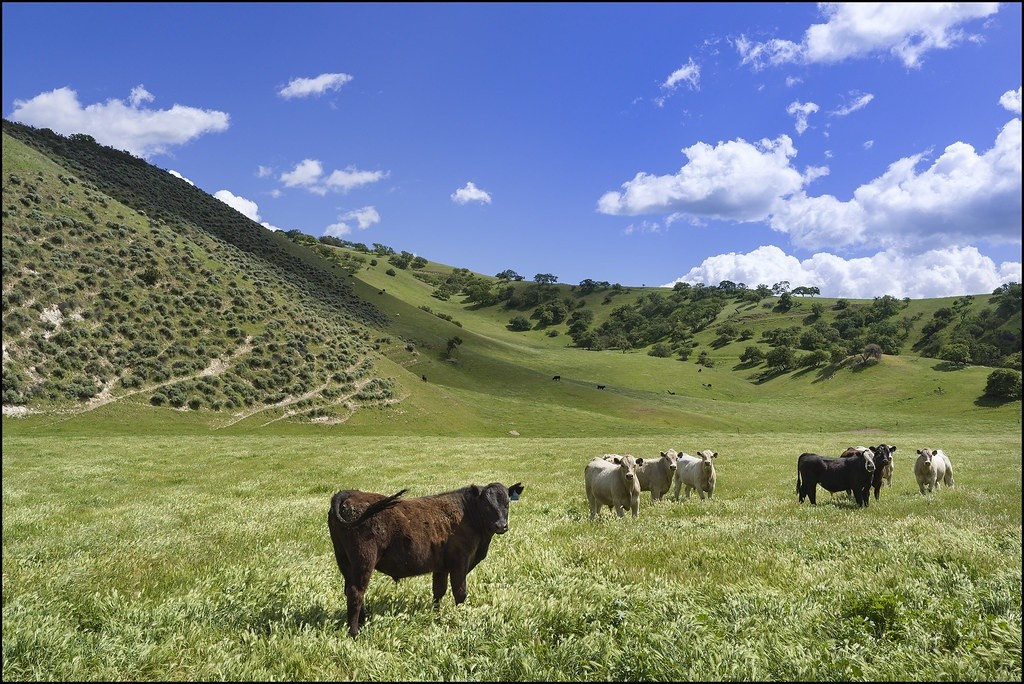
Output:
[327,481,525,636]
[796,443,897,506]
[584,449,718,517]
[597,384,605,390]
[914,447,953,496]
[422,375,427,381]
[553,376,560,381]
